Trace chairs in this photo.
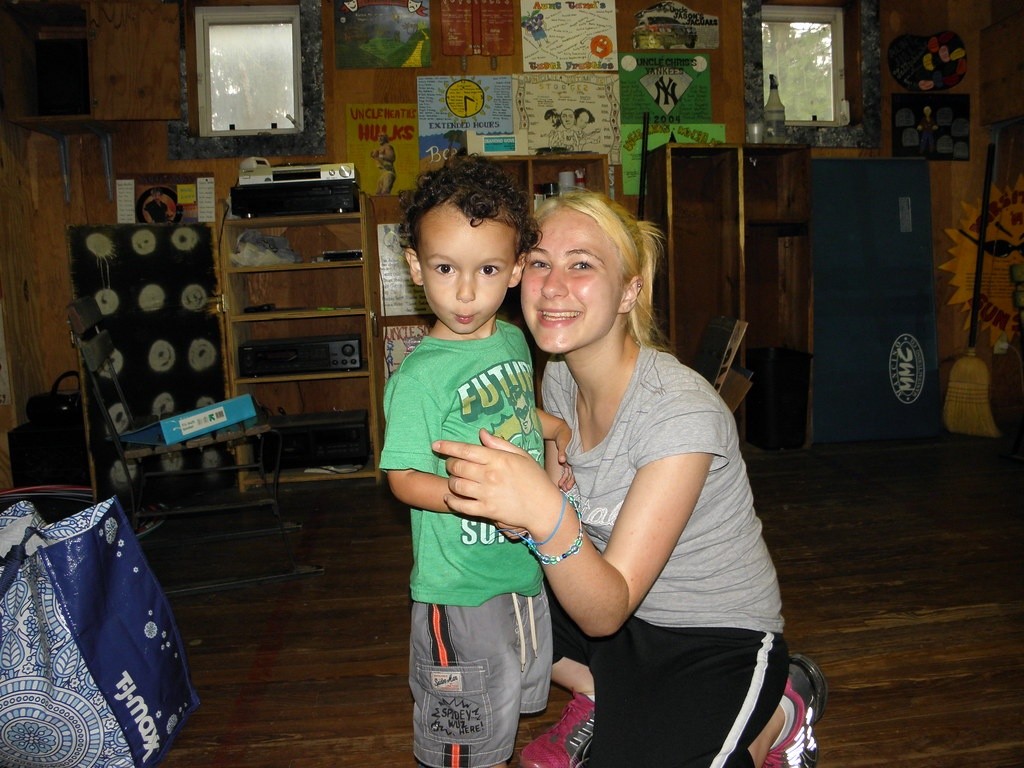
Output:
[63,293,332,599]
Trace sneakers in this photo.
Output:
[758,654,828,768]
[520,686,595,768]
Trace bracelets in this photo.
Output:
[490,489,583,566]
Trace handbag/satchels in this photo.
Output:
[0,495,201,768]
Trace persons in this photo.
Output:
[378,152,575,768]
[433,184,827,768]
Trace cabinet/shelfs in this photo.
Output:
[459,154,609,220]
[219,185,384,494]
[645,141,812,455]
[8,0,185,135]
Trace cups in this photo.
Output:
[747,122,763,143]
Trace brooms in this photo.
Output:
[942,142,1007,437]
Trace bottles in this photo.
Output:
[533,168,585,213]
[764,75,785,143]
